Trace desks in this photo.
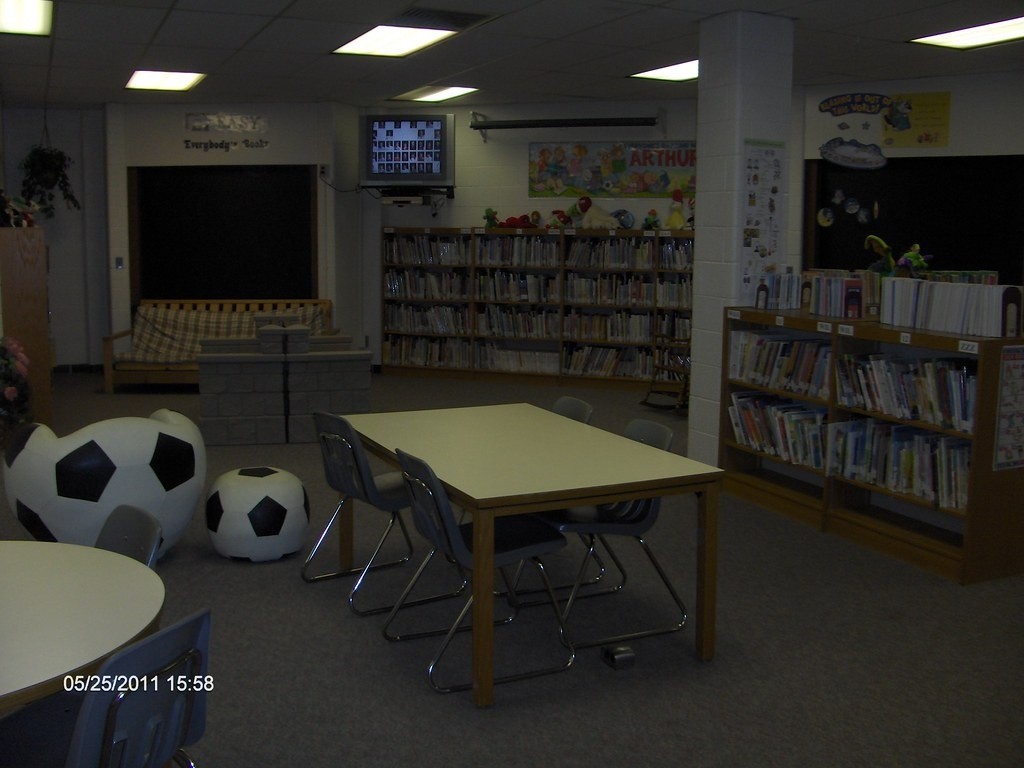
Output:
[337,403,727,709]
[0,539,165,725]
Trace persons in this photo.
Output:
[757,279,769,294]
[372,120,441,173]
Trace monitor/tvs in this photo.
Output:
[358,113,455,188]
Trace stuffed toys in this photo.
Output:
[498,215,537,228]
[904,243,928,268]
[546,197,620,230]
[483,208,497,227]
[642,209,660,230]
[664,201,686,230]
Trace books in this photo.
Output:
[727,269,1024,513]
[383,235,693,382]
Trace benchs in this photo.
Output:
[103,300,332,391]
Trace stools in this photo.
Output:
[205,466,309,565]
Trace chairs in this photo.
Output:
[301,408,466,616]
[0,605,212,768]
[381,448,576,693]
[493,396,605,595]
[533,419,688,648]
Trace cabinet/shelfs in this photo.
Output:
[0,227,50,429]
[718,306,1024,584]
[381,226,694,384]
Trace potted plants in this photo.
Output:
[16,144,82,218]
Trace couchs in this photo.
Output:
[3,408,207,563]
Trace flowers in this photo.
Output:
[0,189,40,227]
[0,336,34,427]
